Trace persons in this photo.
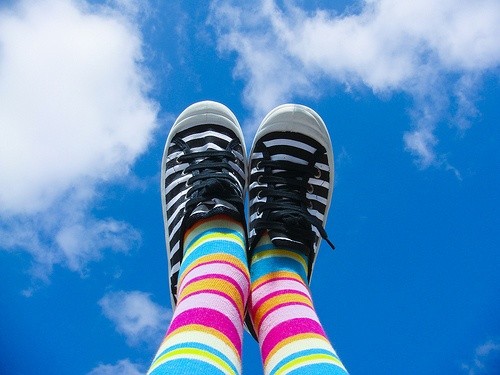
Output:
[141,102,349,373]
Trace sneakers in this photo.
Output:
[242,103,336,340]
[161,99,250,320]
